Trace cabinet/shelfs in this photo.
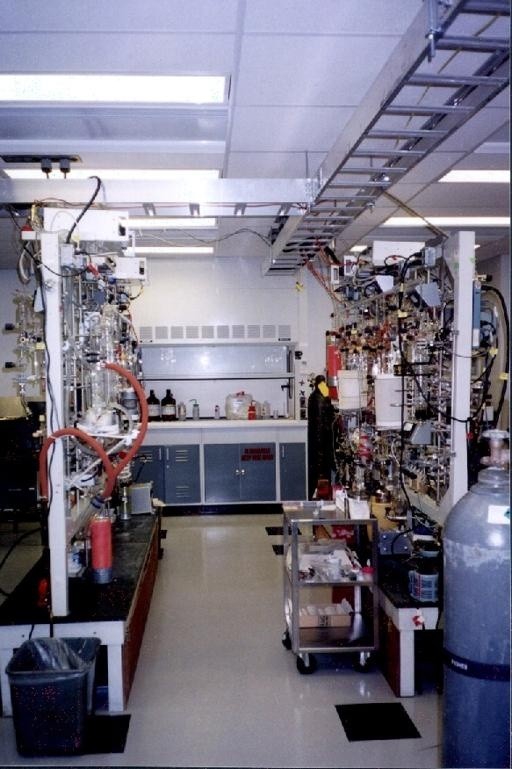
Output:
[282,500,379,675]
[133,442,306,505]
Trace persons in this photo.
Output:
[306,373,334,501]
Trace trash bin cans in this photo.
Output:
[5,637,101,758]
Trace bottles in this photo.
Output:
[247,400,279,421]
[160,388,176,421]
[192,404,200,420]
[177,401,186,421]
[214,405,220,420]
[146,389,162,421]
